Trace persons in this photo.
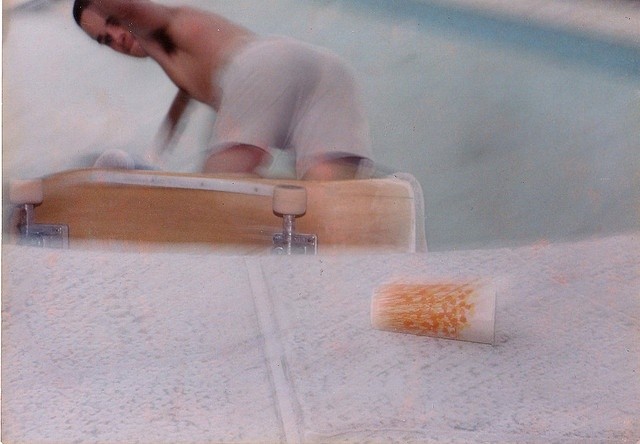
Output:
[73,0,377,182]
